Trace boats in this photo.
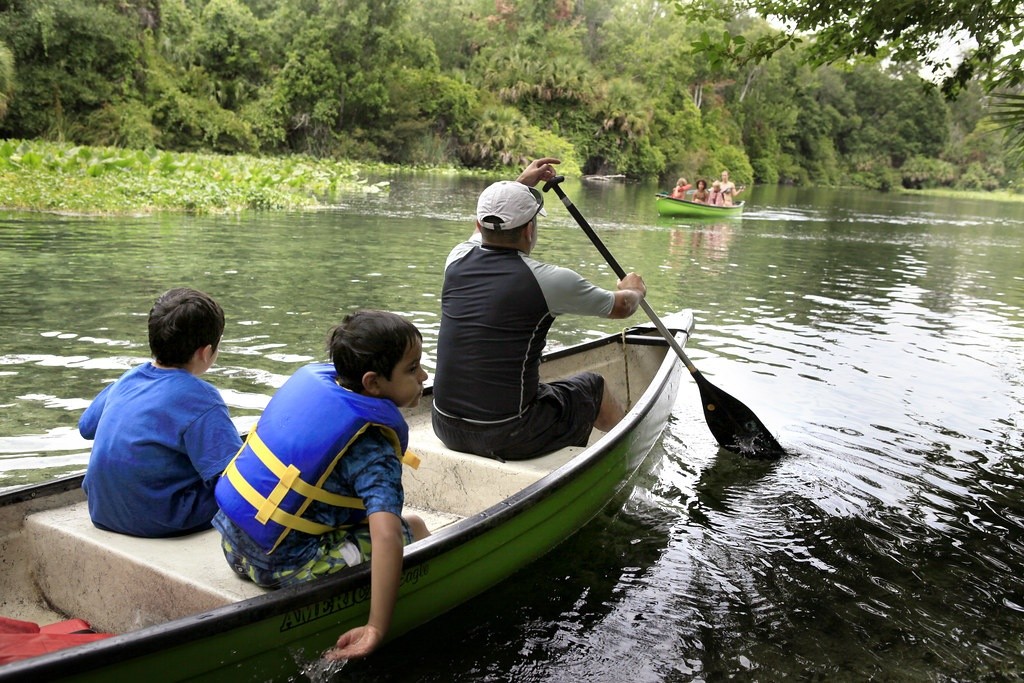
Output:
[653,193,746,216]
[0,310,695,683]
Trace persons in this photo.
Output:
[709,181,725,207]
[210,310,431,658]
[670,177,692,200]
[692,179,708,205]
[78,287,244,539]
[432,158,647,463]
[720,170,743,207]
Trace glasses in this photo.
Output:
[522,185,544,230]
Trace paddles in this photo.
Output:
[542,175,783,449]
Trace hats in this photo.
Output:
[477,180,548,232]
